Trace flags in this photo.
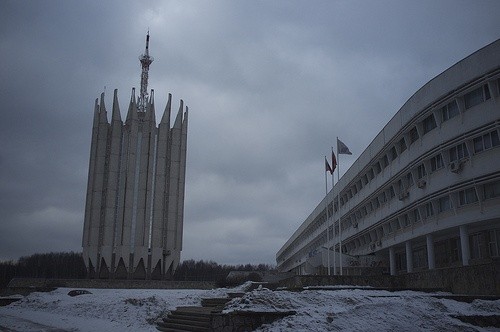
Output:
[337,138,353,156]
[332,150,338,172]
[325,159,333,175]
